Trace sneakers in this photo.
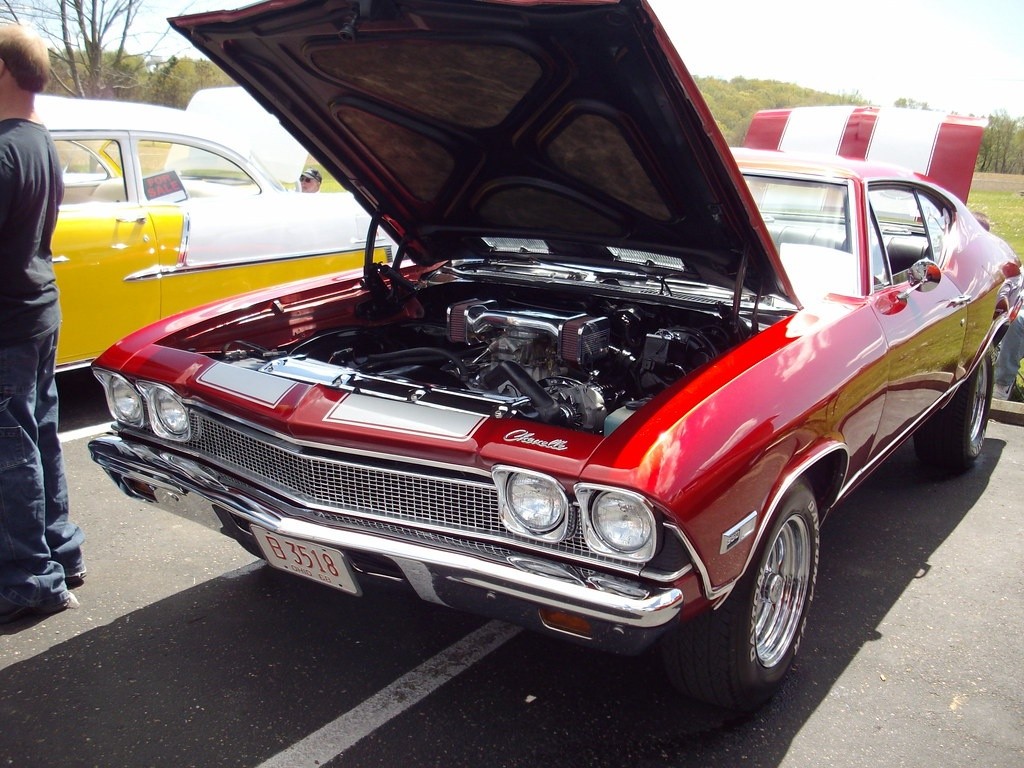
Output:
[1,569,88,624]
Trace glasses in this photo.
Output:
[300,177,314,183]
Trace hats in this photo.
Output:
[301,168,322,183]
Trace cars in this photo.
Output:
[33,85,412,379]
[85,0,1024,717]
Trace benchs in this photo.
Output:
[766,223,930,276]
[91,177,226,202]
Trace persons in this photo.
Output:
[992,264,1024,319]
[990,306,1024,400]
[299,168,324,194]
[0,19,88,629]
